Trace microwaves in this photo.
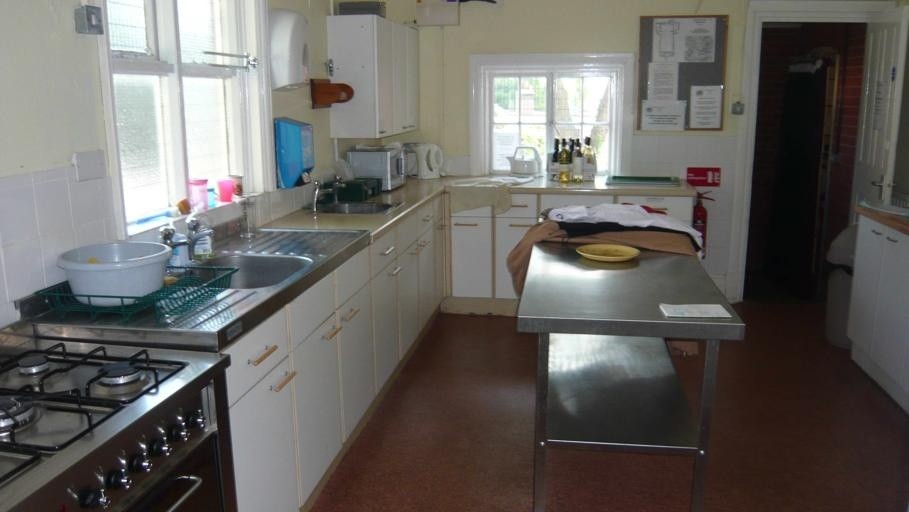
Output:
[349,146,407,192]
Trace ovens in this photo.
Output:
[120,432,236,511]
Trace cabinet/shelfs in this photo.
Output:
[496,184,537,299]
[540,191,701,224]
[369,223,399,399]
[286,237,374,512]
[844,215,908,415]
[401,192,446,370]
[217,307,302,512]
[445,184,494,299]
[326,11,420,140]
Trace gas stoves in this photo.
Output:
[1,340,231,509]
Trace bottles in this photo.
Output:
[549,137,598,186]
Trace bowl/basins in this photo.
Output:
[58,242,173,303]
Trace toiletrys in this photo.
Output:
[185,204,217,261]
[157,216,190,274]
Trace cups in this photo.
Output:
[185,174,236,212]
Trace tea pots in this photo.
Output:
[505,144,546,178]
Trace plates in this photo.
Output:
[576,244,642,262]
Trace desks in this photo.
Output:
[514,230,748,512]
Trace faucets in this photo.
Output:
[332,175,346,203]
[158,226,191,274]
[312,180,334,212]
[186,218,217,261]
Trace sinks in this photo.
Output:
[321,201,393,215]
[173,254,314,291]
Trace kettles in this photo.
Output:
[410,141,443,181]
